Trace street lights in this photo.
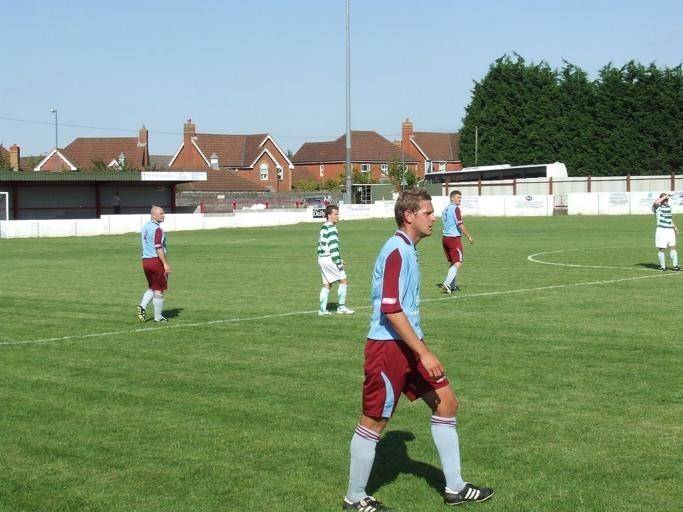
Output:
[50,109,60,155]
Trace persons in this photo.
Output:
[342,191,492,512]
[438,191,473,295]
[317,204,354,317]
[652,193,681,272]
[114,191,121,214]
[137,207,171,323]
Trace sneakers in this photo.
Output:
[440,282,453,295]
[658,267,666,271]
[336,308,355,315]
[137,305,146,323]
[154,317,167,323]
[450,286,462,291]
[444,483,495,505]
[673,267,680,271]
[318,310,332,315]
[342,496,386,512]
